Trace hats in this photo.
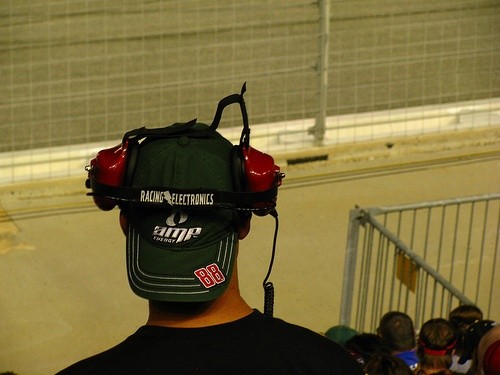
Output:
[120,122,252,301]
[323,325,359,345]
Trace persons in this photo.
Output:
[326,302,500,375]
[54,121,365,375]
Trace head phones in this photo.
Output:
[84,82,286,217]
